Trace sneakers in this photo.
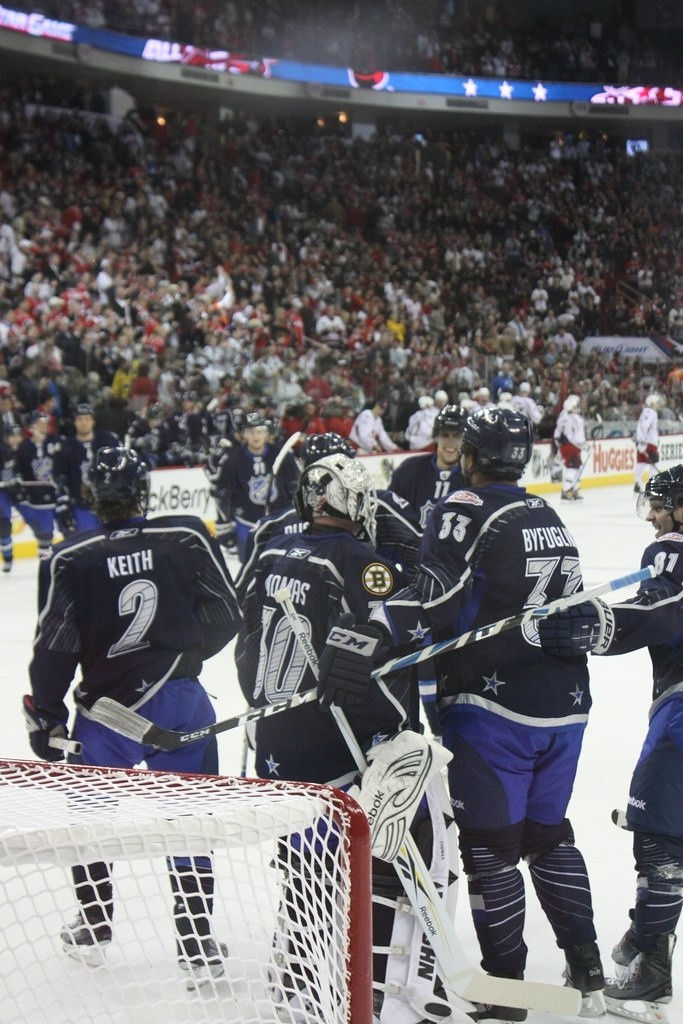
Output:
[612,908,647,975]
[174,902,227,992]
[469,946,527,1024]
[561,943,607,1018]
[602,933,676,1024]
[60,885,116,968]
[562,488,583,504]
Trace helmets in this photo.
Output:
[644,462,683,509]
[239,411,271,434]
[294,453,379,547]
[4,383,661,436]
[84,446,150,514]
[461,407,533,481]
[432,406,471,442]
[299,432,355,465]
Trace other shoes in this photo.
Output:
[634,482,645,494]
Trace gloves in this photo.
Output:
[537,595,614,657]
[24,694,69,764]
[315,617,385,714]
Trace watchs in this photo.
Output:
[22,444,246,991]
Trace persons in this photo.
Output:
[235,453,433,1024]
[1,1,682,733]
[539,462,683,1024]
[317,405,607,1024]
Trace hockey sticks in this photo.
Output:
[90,563,658,751]
[48,737,83,756]
[270,587,586,1015]
